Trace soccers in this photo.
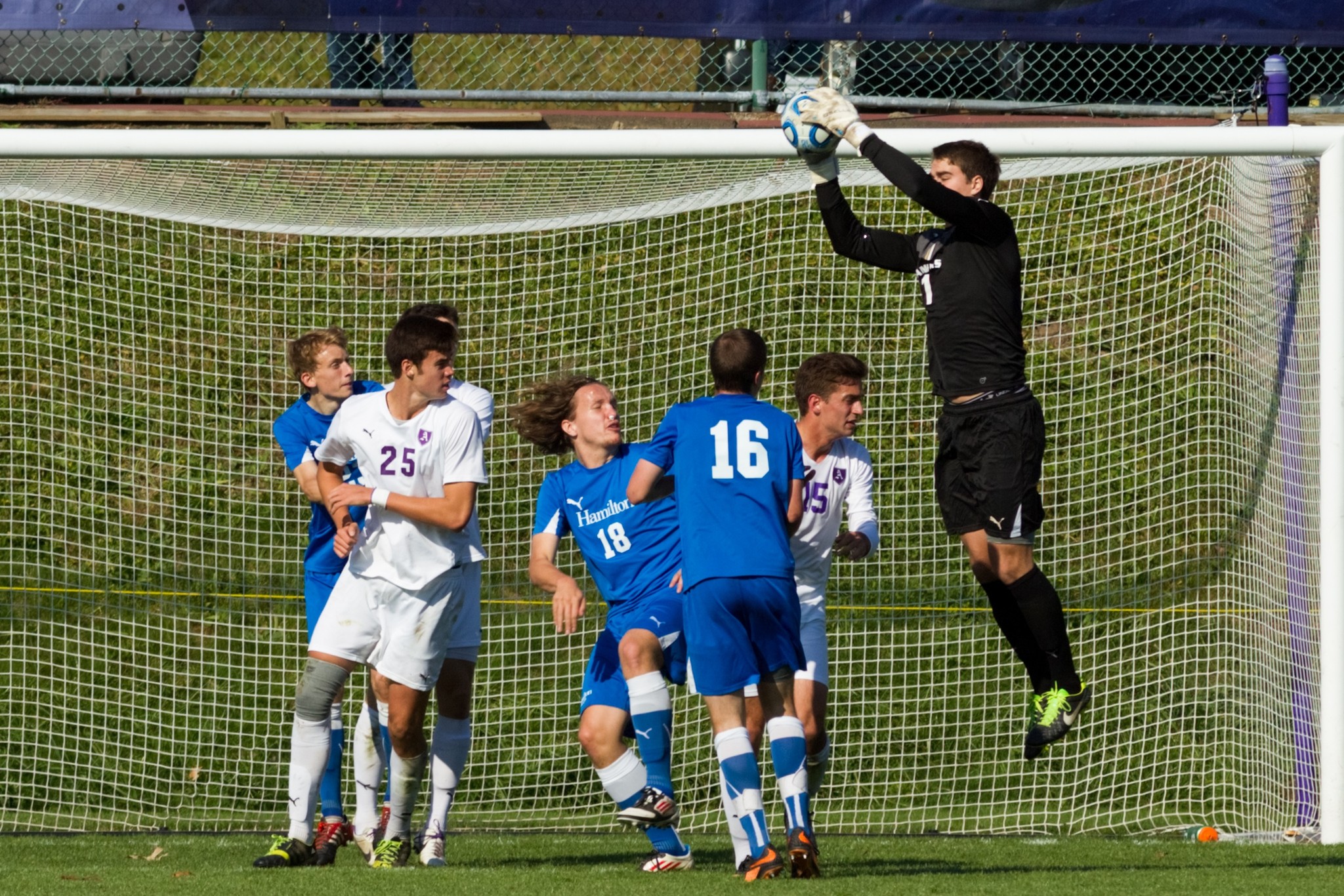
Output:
[780,89,843,157]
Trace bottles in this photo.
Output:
[1263,54,1289,126]
[1183,826,1218,843]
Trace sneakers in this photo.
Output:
[786,828,822,880]
[806,745,830,800]
[374,806,391,841]
[310,814,352,867]
[636,844,694,872]
[615,788,680,829]
[414,819,456,867]
[369,837,411,868]
[743,844,784,882]
[1037,678,1091,744]
[252,834,311,869]
[1024,697,1053,766]
[352,819,382,862]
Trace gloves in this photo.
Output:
[798,153,841,185]
[800,87,875,160]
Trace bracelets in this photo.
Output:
[371,487,390,510]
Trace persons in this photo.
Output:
[627,328,824,882]
[784,85,1090,761]
[513,376,695,873]
[745,352,878,859]
[254,302,496,868]
[323,32,420,109]
